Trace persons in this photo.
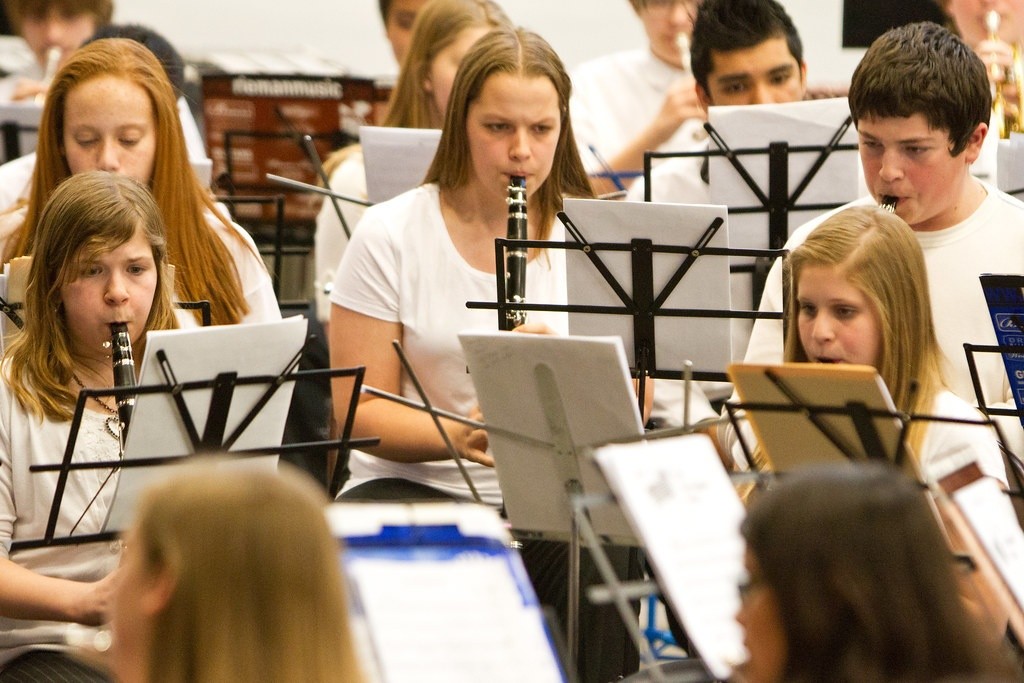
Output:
[0,0,285,323]
[727,462,1024,681]
[0,171,186,683]
[311,0,1024,683]
[111,457,364,681]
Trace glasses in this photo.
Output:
[733,566,771,606]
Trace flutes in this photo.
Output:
[504,171,528,342]
[106,321,145,453]
